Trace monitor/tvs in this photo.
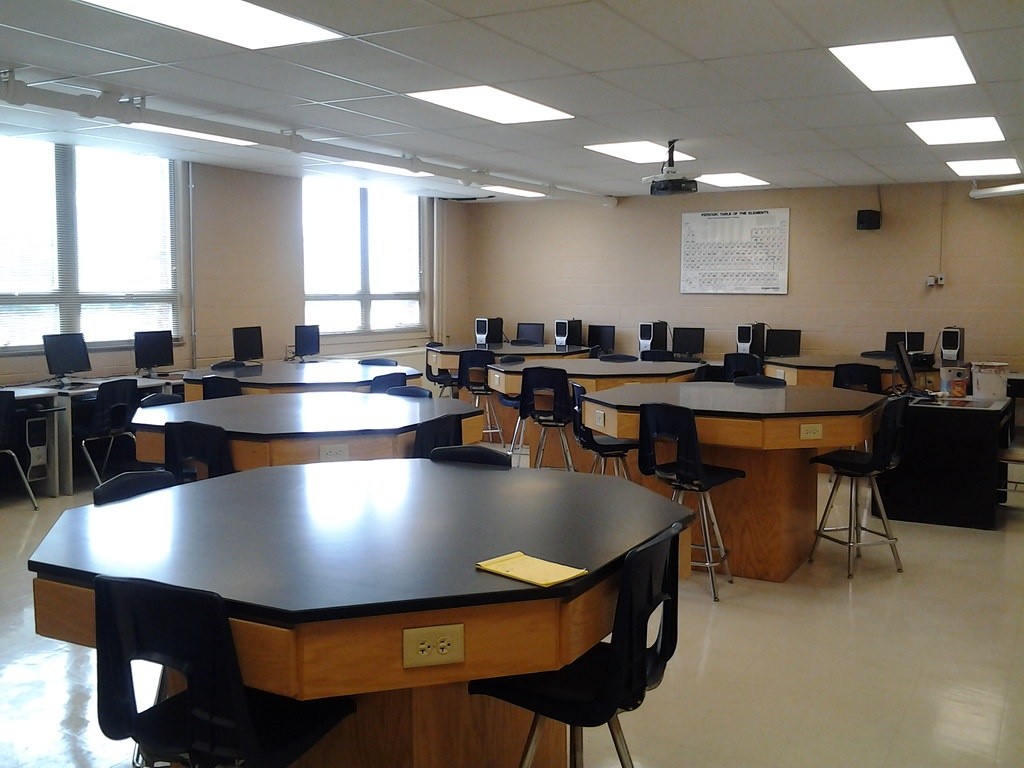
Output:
[517,323,544,344]
[587,325,615,349]
[134,330,174,373]
[671,327,705,357]
[233,326,264,361]
[43,333,92,378]
[885,332,922,352]
[766,330,801,356]
[294,325,319,356]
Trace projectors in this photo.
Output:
[650,180,697,196]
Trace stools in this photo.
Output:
[999,446,1024,514]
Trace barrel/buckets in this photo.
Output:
[939,367,966,398]
[971,361,1010,398]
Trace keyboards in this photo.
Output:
[673,357,701,363]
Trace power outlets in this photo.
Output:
[595,411,606,427]
[920,377,934,386]
[318,445,351,462]
[800,425,824,440]
[403,622,465,669]
[494,374,501,386]
[432,355,437,363]
[776,370,785,379]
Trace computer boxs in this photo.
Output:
[475,317,503,344]
[736,323,765,354]
[941,328,964,361]
[639,321,668,351]
[554,320,582,346]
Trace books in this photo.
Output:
[475,550,589,588]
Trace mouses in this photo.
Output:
[701,361,706,364]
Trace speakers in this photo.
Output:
[857,210,881,230]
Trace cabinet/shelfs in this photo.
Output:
[906,398,1015,529]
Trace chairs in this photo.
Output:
[0,349,906,766]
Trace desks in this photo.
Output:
[487,360,705,445]
[132,388,486,478]
[760,356,972,397]
[181,359,421,401]
[425,347,591,407]
[581,380,888,582]
[27,457,695,768]
[1,387,60,498]
[1006,372,1024,440]
[30,372,185,496]
